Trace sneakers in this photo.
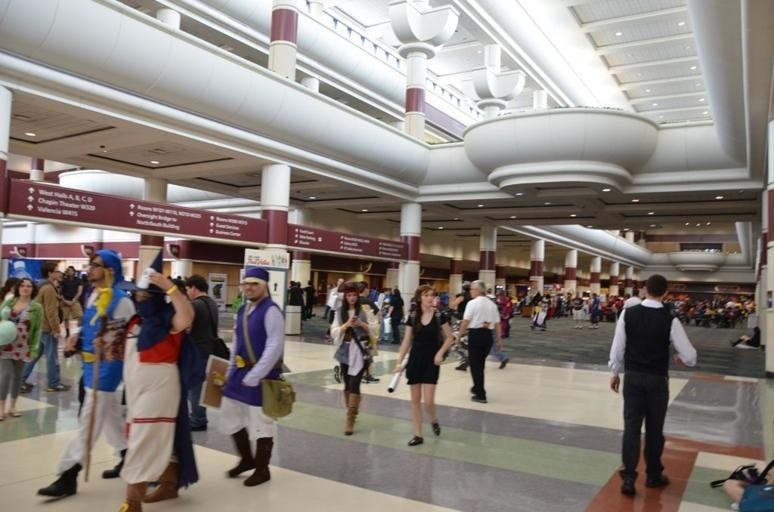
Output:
[621,483,636,495]
[499,357,509,369]
[19,381,33,393]
[408,435,424,445]
[189,414,207,428]
[46,382,71,392]
[334,365,343,384]
[646,475,670,487]
[455,364,467,369]
[470,385,486,403]
[431,421,441,436]
[361,375,380,384]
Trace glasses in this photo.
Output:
[88,261,105,268]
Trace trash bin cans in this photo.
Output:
[283,305,302,335]
[747,314,758,329]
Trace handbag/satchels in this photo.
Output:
[730,462,768,485]
[260,377,297,418]
[363,347,373,368]
[438,339,450,361]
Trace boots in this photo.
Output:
[344,390,361,435]
[243,436,273,486]
[36,462,82,497]
[143,462,179,503]
[228,427,256,478]
[103,448,127,478]
[117,482,148,512]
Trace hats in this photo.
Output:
[97,249,123,285]
[242,268,269,285]
[338,272,369,293]
[114,248,167,295]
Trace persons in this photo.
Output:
[321,278,509,447]
[722,460,774,512]
[608,274,697,497]
[1,249,290,511]
[488,279,647,338]
[663,292,774,349]
[287,279,316,320]
